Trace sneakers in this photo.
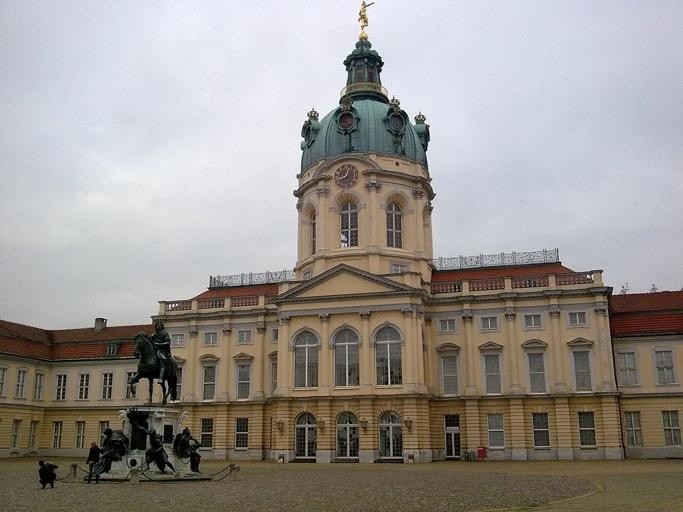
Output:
[87,481,99,484]
[50,486,55,489]
[41,485,46,490]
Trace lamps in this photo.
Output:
[276,419,284,430]
[360,416,369,428]
[317,418,325,429]
[404,416,413,428]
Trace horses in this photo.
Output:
[129,331,179,404]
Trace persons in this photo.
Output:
[84,440,101,484]
[147,320,172,385]
[173,427,202,474]
[357,1,375,32]
[136,424,179,476]
[36,459,59,489]
[86,426,128,476]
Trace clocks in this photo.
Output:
[333,164,360,189]
